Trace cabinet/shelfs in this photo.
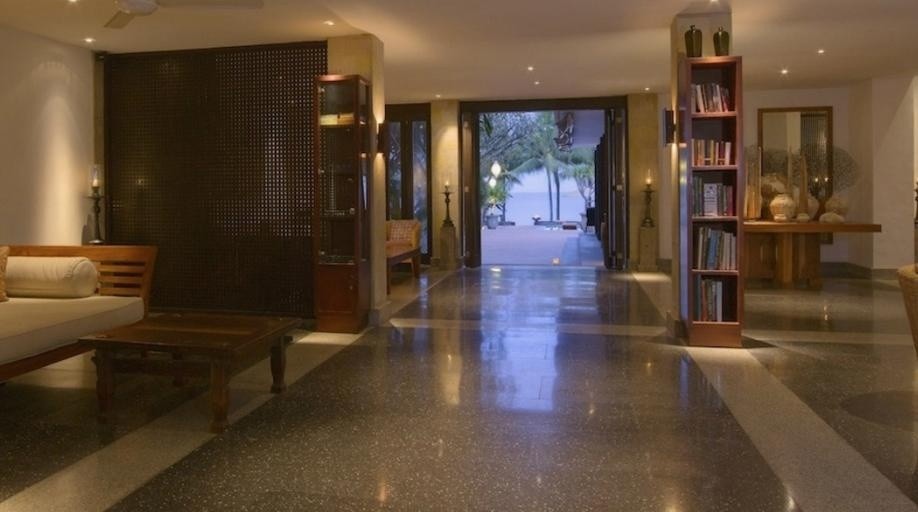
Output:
[312,75,375,333]
[677,56,744,347]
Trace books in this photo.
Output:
[696,226,737,272]
[694,277,729,323]
[690,171,737,216]
[689,82,734,113]
[690,137,736,167]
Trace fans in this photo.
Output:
[104,0,264,29]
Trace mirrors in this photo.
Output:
[757,106,833,202]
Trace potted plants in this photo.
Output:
[559,147,595,231]
[480,160,523,229]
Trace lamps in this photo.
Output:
[85,169,105,245]
[638,168,659,272]
[439,180,458,271]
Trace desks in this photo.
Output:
[743,219,881,291]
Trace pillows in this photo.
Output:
[0,246,8,302]
[6,255,99,298]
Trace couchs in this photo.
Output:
[0,243,159,382]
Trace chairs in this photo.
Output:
[386,219,422,295]
